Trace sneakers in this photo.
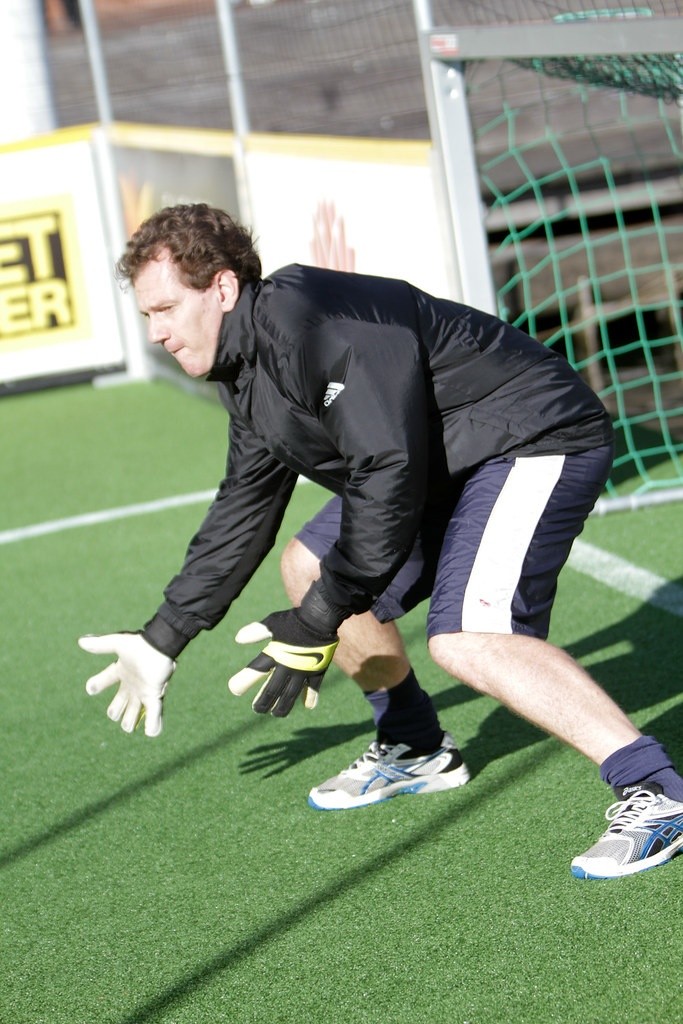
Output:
[309,730,470,810]
[571,780,683,880]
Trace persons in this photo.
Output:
[78,203,683,880]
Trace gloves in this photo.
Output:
[78,613,190,738]
[228,580,345,719]
